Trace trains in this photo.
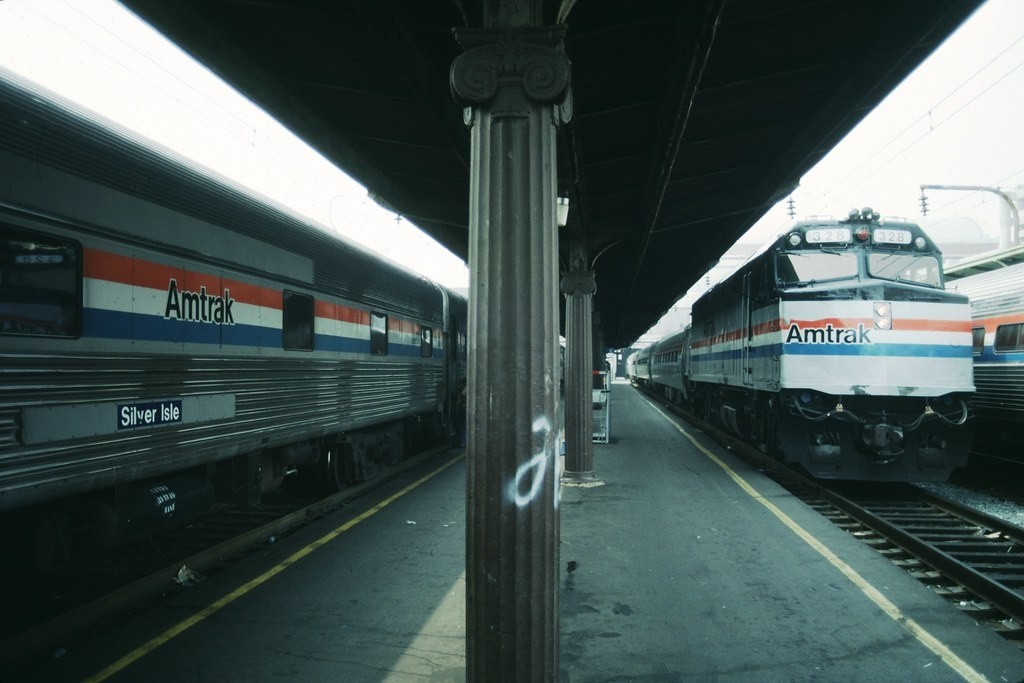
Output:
[943,244,1024,456]
[626,208,976,480]
[1,66,566,575]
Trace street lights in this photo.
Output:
[920,185,1019,246]
